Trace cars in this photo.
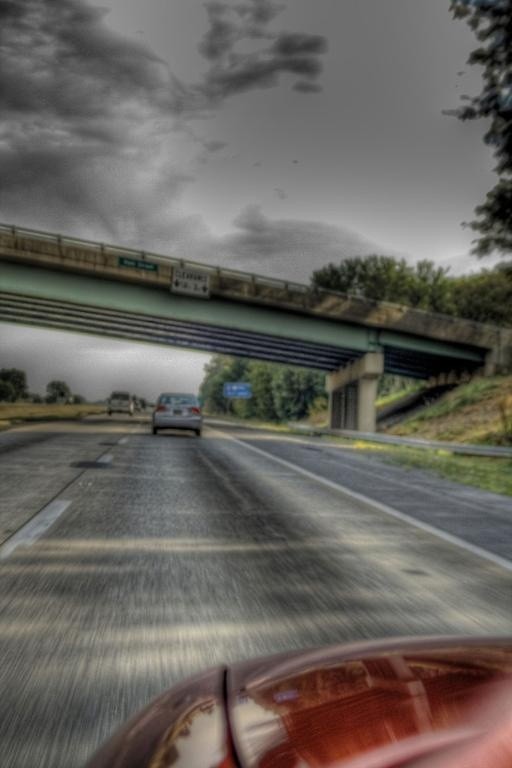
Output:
[152,391,203,437]
[81,634,511,767]
[106,390,155,416]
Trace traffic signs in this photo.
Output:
[170,266,210,298]
[118,256,157,272]
[224,382,252,397]
